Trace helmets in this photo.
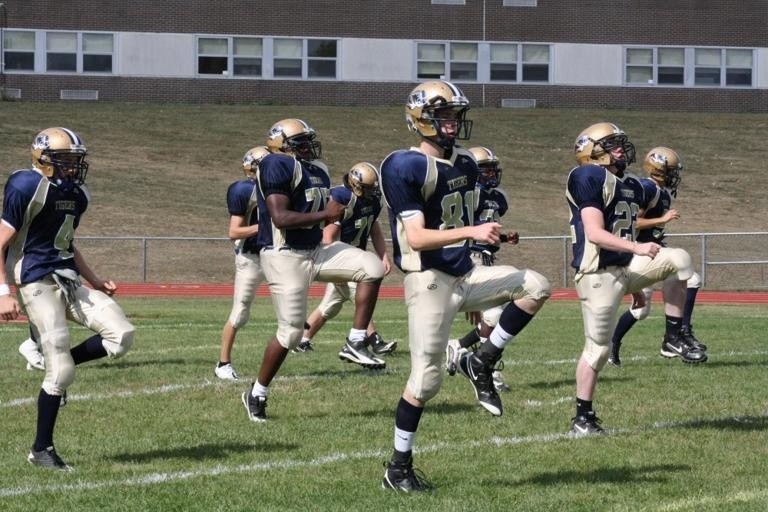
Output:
[29,126,88,179]
[643,145,683,187]
[346,160,382,200]
[574,121,628,168]
[265,116,316,159]
[404,79,470,140]
[242,146,269,181]
[468,146,499,167]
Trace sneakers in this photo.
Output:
[569,409,610,438]
[607,340,623,369]
[290,340,314,354]
[17,336,69,407]
[680,326,707,351]
[213,361,240,382]
[240,382,267,425]
[381,455,429,495]
[453,346,512,418]
[25,443,68,471]
[659,334,709,365]
[446,339,468,377]
[338,333,386,371]
[372,334,398,356]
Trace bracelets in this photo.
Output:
[0,284,12,299]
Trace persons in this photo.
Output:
[600,145,707,368]
[378,75,553,501]
[239,118,389,428]
[286,161,398,359]
[566,120,707,439]
[213,144,312,382]
[0,125,137,474]
[443,143,522,396]
[19,323,48,371]
